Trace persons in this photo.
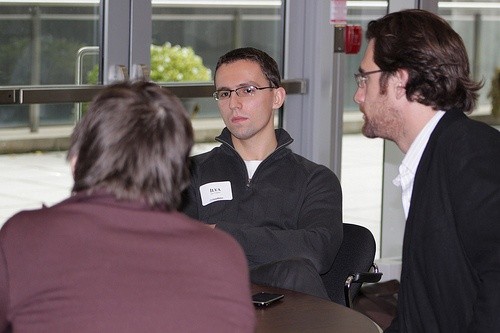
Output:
[354,9,500,333]
[0,82,255,333]
[177,47,342,300]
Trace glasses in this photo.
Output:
[213,86,273,101]
[354,69,381,86]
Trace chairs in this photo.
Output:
[320,223,376,305]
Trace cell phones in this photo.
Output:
[252,292,284,308]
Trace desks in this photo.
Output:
[250,282,384,333]
